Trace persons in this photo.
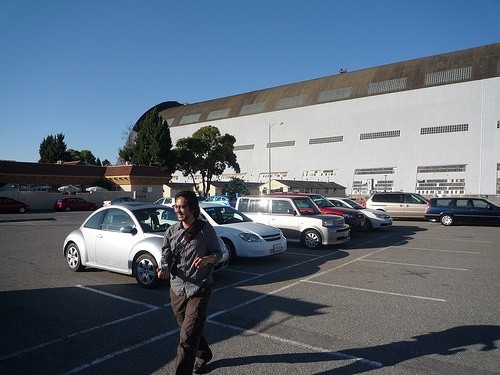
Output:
[155,191,224,375]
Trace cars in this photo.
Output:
[326,197,393,233]
[202,196,236,207]
[56,197,98,211]
[133,201,288,264]
[153,197,176,208]
[109,197,135,204]
[63,201,231,289]
[0,196,30,213]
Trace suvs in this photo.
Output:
[269,192,365,233]
[236,195,352,249]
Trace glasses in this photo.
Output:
[172,204,190,210]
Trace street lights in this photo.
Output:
[268,122,284,194]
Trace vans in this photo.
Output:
[366,192,441,222]
[423,196,500,226]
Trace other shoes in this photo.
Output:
[192,349,213,374]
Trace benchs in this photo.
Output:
[107,215,129,230]
